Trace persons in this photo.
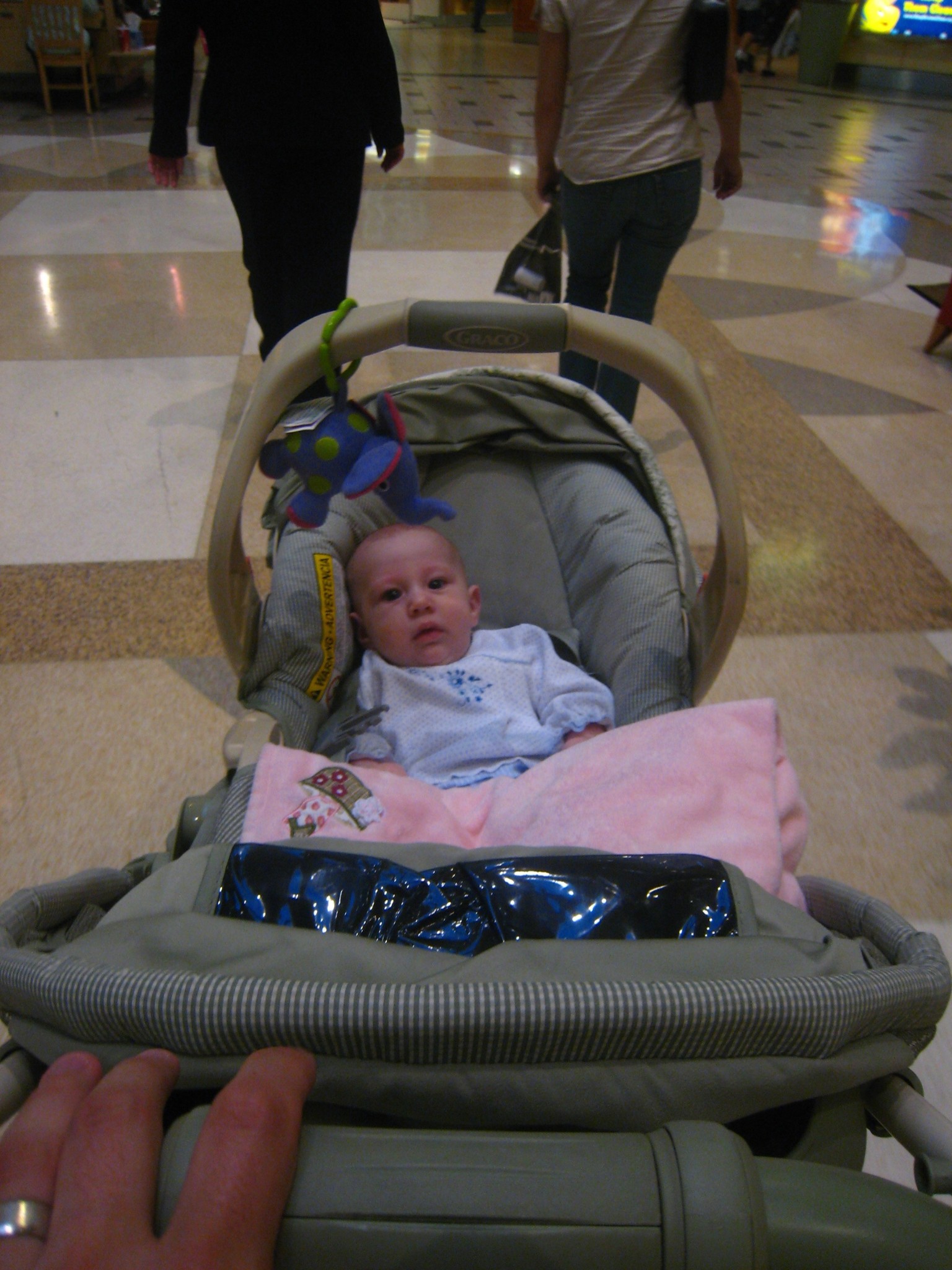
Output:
[146,0,405,399]
[24,0,100,113]
[534,0,796,423]
[344,523,616,789]
[472,0,486,34]
[0,1047,318,1270]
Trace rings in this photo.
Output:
[0,1199,52,1243]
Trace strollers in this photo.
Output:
[1,292,950,1270]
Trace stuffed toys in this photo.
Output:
[252,377,458,529]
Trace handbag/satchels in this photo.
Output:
[494,205,563,305]
[683,0,730,105]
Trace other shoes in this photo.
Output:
[736,57,754,73]
[762,69,775,77]
